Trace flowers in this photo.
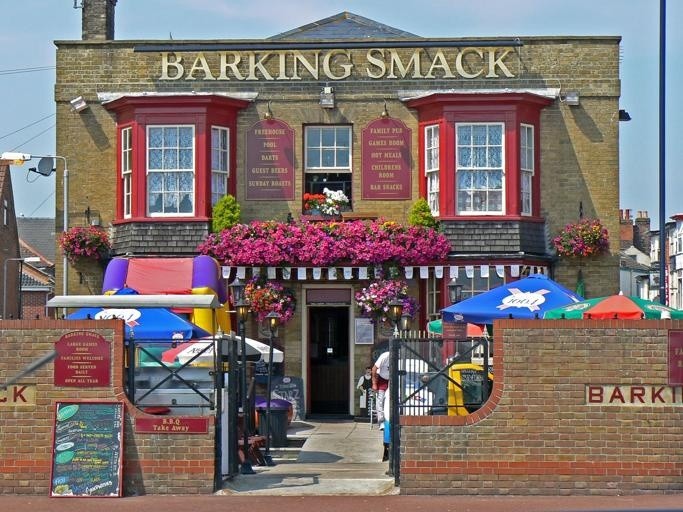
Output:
[303,186,349,213]
[239,272,296,325]
[56,225,111,266]
[552,219,609,261]
[354,270,420,326]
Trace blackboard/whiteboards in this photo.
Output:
[271,376,304,421]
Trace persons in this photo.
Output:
[372,351,390,431]
[356,366,373,417]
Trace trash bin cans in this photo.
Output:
[255,399,292,446]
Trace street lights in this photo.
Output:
[229,276,244,338]
[399,312,411,416]
[232,297,257,474]
[2,255,43,319]
[0,149,71,321]
[387,298,402,337]
[262,311,280,468]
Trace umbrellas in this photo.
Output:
[439,269,587,327]
[542,290,683,321]
[424,318,493,338]
[64,285,213,349]
[160,332,286,373]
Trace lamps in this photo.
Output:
[380,101,389,119]
[263,99,273,120]
